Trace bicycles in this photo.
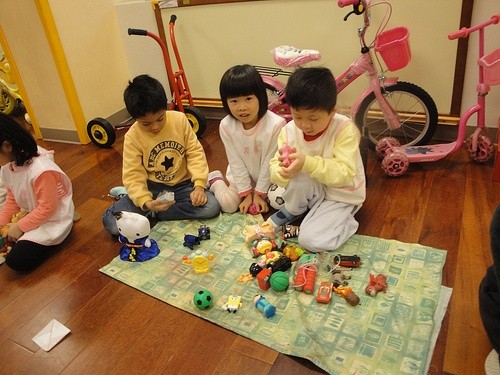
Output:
[254,0,438,152]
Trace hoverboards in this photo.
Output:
[376,14,500,178]
[87,15,206,148]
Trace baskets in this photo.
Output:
[479,48,500,86]
[374,26,412,72]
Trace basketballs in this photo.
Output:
[269,271,289,292]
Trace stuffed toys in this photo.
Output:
[112,210,160,262]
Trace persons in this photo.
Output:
[0,112,81,273]
[207,64,287,213]
[102,74,220,236]
[479,204,500,365]
[265,66,366,253]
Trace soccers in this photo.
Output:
[267,183,287,210]
[193,289,214,310]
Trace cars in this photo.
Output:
[334,253,360,267]
[315,280,333,304]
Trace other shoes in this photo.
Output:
[351,204,363,216]
[206,170,223,189]
[107,186,128,199]
[266,206,302,228]
